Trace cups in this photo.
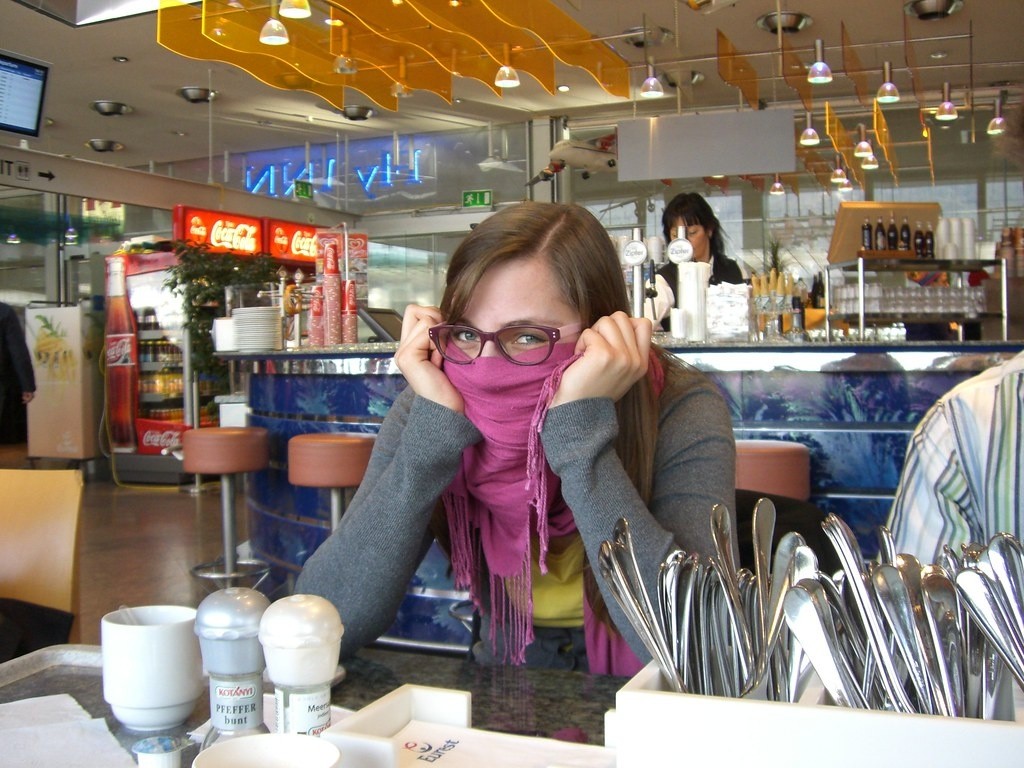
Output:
[100,606,203,727]
[670,260,713,339]
[1001,227,1024,278]
[835,283,986,312]
[807,321,906,342]
[309,244,360,347]
[191,732,341,768]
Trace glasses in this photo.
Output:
[428,320,582,366]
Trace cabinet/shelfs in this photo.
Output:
[822,257,1010,340]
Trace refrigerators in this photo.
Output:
[104,252,220,487]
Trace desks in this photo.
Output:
[327,646,642,746]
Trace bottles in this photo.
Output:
[812,272,824,308]
[749,319,788,345]
[937,217,977,259]
[135,307,213,423]
[104,258,142,441]
[793,278,809,309]
[863,216,933,258]
[751,267,793,297]
[785,314,811,343]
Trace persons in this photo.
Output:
[280,200,741,677]
[874,344,1024,566]
[639,191,748,334]
[0,304,36,445]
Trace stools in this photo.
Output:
[286,430,379,532]
[731,439,812,503]
[182,425,273,593]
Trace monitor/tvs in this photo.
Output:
[0,48,54,142]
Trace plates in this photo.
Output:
[214,307,283,349]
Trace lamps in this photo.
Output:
[7,0,1006,246]
